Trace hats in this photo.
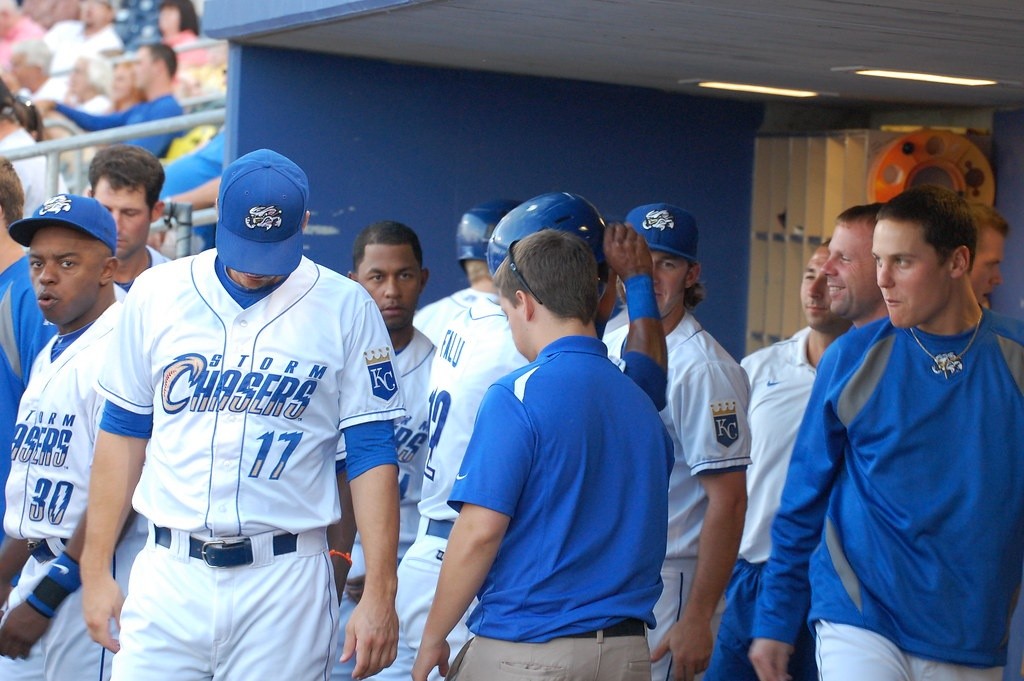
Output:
[626,204,698,262]
[8,194,116,255]
[216,148,309,276]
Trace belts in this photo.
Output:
[27,539,69,563]
[561,618,646,637]
[426,518,455,540]
[154,524,299,567]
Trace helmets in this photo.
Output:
[457,200,523,262]
[487,192,609,301]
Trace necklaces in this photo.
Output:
[909,314,983,379]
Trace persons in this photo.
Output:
[748,185,1024,681]
[967,203,1010,307]
[0,193,148,681]
[156,0,206,83]
[410,228,675,681]
[704,236,854,681]
[33,0,125,101]
[326,221,438,681]
[0,0,228,260]
[79,148,398,681]
[85,144,173,303]
[821,202,889,327]
[600,203,752,681]
[412,198,523,349]
[0,154,60,610]
[486,191,667,412]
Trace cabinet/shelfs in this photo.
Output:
[747,127,907,356]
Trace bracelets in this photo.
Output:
[26,551,81,619]
[330,550,352,566]
[624,276,660,323]
